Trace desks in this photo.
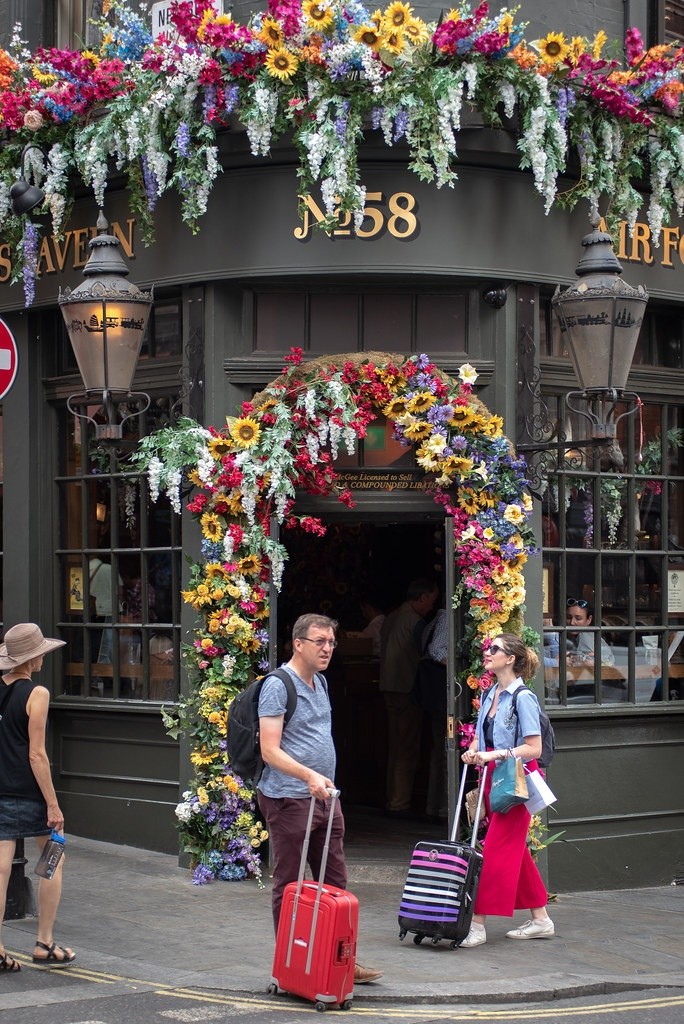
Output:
[65,662,175,680]
[544,664,684,698]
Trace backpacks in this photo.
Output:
[227,671,330,787]
[481,684,556,768]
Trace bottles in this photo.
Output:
[34,828,65,879]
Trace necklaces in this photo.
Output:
[9,672,32,681]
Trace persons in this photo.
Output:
[544,598,615,668]
[461,632,555,948]
[148,618,173,700]
[258,614,384,984]
[87,553,125,689]
[356,583,449,824]
[0,623,76,973]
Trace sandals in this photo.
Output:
[33,941,76,963]
[0,954,23,972]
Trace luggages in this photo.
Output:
[398,760,488,950]
[265,787,359,1011]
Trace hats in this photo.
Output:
[0,623,67,670]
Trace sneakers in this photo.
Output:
[505,920,555,940]
[458,921,487,947]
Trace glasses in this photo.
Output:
[566,598,589,607]
[486,645,511,655]
[299,635,339,648]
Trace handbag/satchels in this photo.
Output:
[522,764,558,815]
[489,750,529,814]
[465,787,486,828]
[410,617,446,713]
[89,596,97,617]
[119,601,133,636]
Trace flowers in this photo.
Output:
[0,0,684,308]
[134,348,581,887]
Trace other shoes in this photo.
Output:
[427,813,448,826]
[384,809,423,819]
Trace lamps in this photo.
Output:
[512,204,650,498]
[58,211,157,445]
[9,144,57,215]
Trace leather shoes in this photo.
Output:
[353,963,384,982]
